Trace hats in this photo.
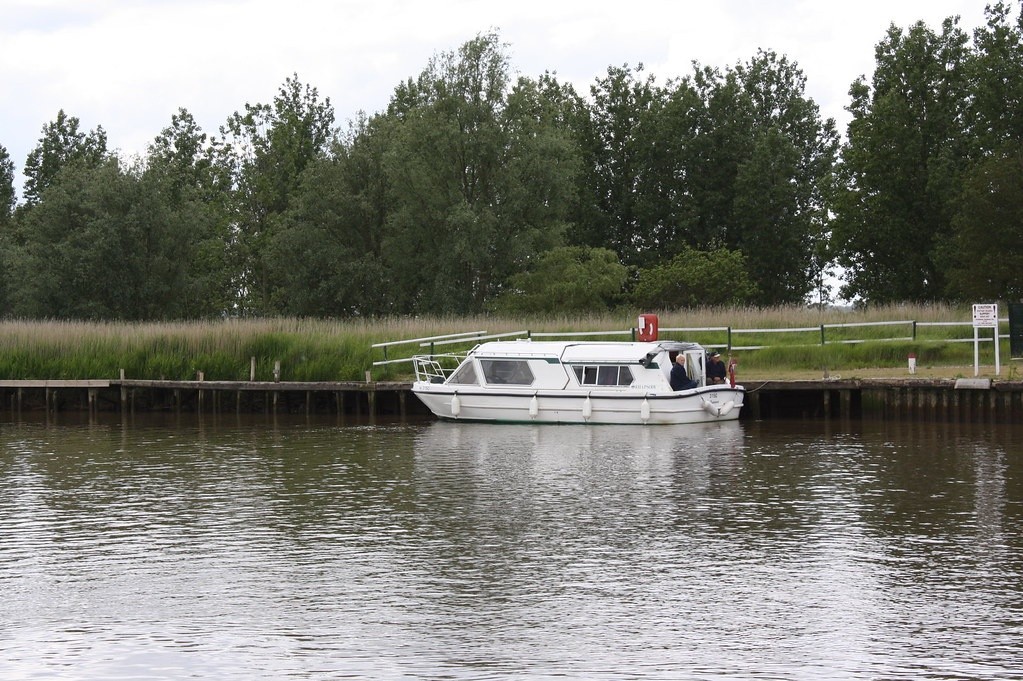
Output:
[711,352,720,357]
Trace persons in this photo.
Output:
[670,354,700,391]
[706,352,726,385]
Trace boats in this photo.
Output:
[411,339,747,425]
